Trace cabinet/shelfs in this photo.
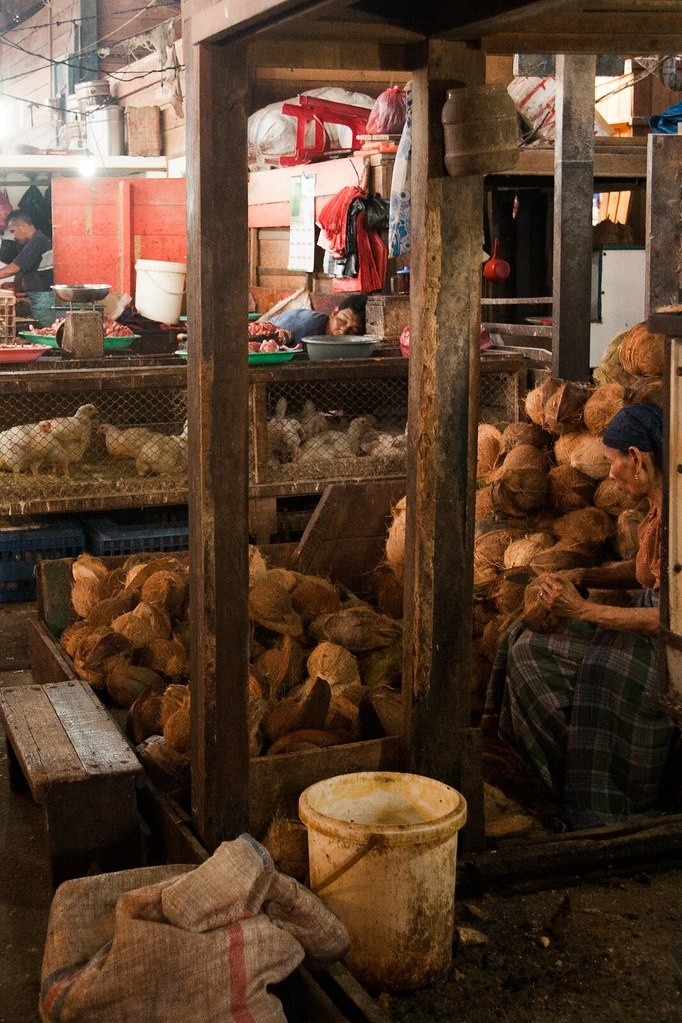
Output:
[247,151,404,341]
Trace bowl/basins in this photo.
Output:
[300,335,379,362]
[50,284,111,303]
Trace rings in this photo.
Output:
[540,593,544,597]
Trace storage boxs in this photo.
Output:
[507,76,616,143]
[0,514,192,606]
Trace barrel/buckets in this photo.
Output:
[59,79,124,157]
[299,770,468,992]
[134,258,186,326]
[441,82,520,177]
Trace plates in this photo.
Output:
[179,312,262,326]
[0,331,141,362]
[175,348,303,365]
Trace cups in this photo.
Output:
[396,267,410,295]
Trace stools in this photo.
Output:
[0,677,143,895]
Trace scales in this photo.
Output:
[50,284,111,361]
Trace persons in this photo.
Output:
[478,402,682,829]
[0,209,53,291]
[268,294,368,349]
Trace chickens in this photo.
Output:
[0,395,410,482]
